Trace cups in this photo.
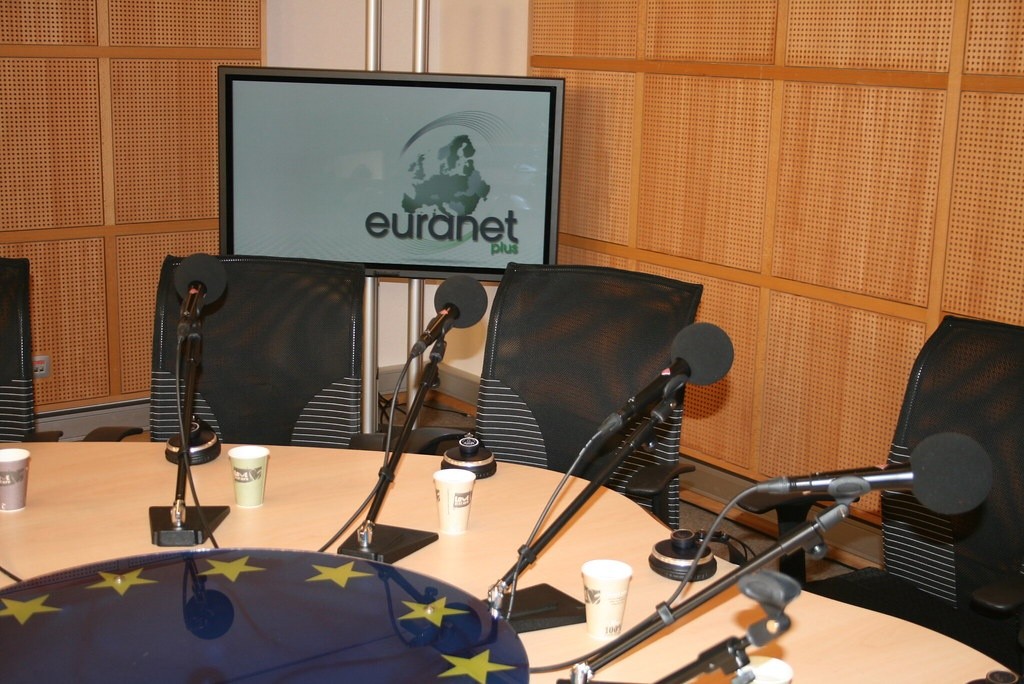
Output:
[433,468,477,535]
[228,445,271,509]
[580,558,633,641]
[0,448,32,513]
[736,656,794,684]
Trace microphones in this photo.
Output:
[599,321,735,438]
[169,252,228,348]
[408,274,488,362]
[753,431,996,516]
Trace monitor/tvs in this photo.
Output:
[216,65,567,284]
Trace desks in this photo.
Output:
[0,439,1019,684]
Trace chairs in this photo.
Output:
[404,257,704,533]
[83,252,387,454]
[739,314,1024,680]
[1,257,66,444]
